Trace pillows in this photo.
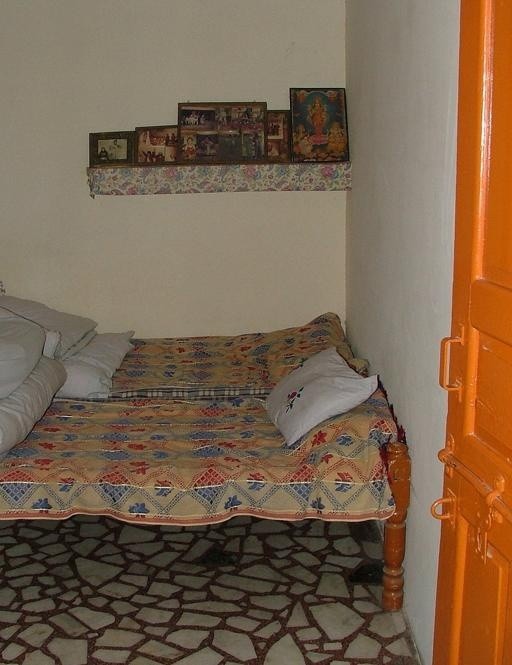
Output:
[262,346,380,447]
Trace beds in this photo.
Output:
[0,369,413,614]
[0,292,369,399]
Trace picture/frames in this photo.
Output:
[134,125,178,165]
[177,101,267,166]
[264,109,292,163]
[88,131,138,168]
[289,87,350,163]
